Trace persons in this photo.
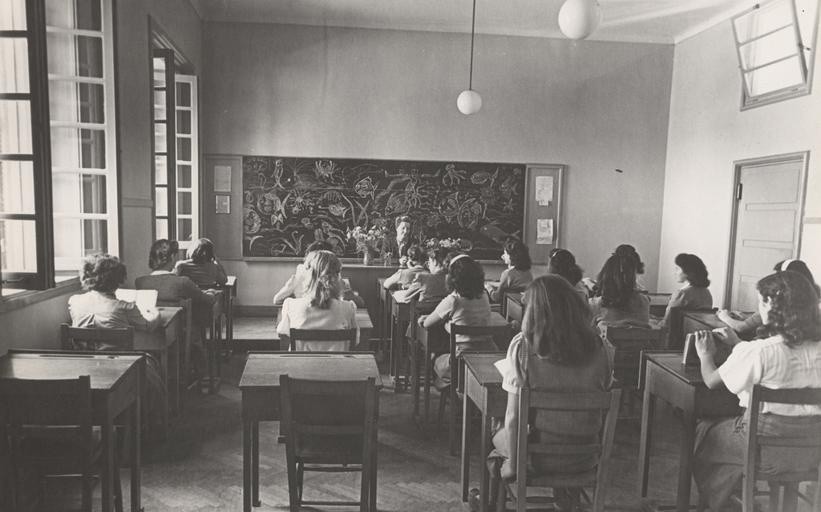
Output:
[470,272,614,512]
[382,244,491,390]
[716,259,821,338]
[175,238,228,290]
[381,215,418,264]
[67,252,161,350]
[547,248,589,302]
[483,234,533,303]
[135,239,216,355]
[649,253,712,348]
[586,244,652,352]
[692,270,821,512]
[273,239,367,351]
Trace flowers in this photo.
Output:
[346,225,390,253]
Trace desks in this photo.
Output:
[237,350,386,512]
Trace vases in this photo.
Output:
[362,250,374,266]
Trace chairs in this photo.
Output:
[278,372,379,512]
[265,265,374,359]
[375,266,816,510]
[4,265,237,512]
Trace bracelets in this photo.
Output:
[205,291,216,296]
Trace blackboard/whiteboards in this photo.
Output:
[241,155,528,265]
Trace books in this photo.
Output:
[682,334,730,364]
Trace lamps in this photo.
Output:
[456,1,483,117]
[559,1,604,45]
